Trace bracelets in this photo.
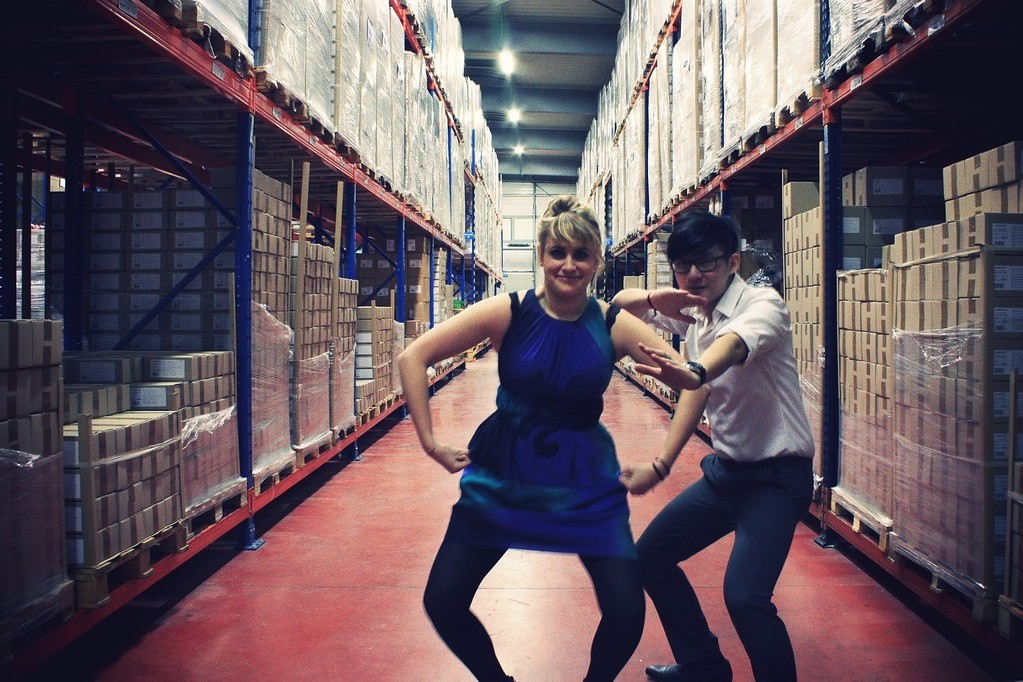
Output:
[426,441,439,455]
[652,456,671,480]
[646,291,656,316]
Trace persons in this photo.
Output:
[397,195,711,682]
[609,210,816,682]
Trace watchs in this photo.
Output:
[685,360,707,390]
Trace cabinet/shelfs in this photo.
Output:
[0,0,472,682]
[465,80,505,362]
[574,0,1023,682]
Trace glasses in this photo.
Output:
[668,254,729,273]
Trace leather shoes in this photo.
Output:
[645,660,733,682]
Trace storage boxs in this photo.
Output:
[572,0,1023,613]
[0,0,507,652]
